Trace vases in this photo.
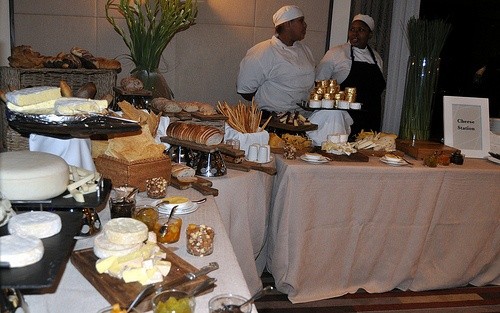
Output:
[399,56,441,142]
[130,65,172,100]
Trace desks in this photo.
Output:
[7,178,260,313]
[266,152,500,304]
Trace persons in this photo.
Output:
[315,14,386,132]
[236,5,317,119]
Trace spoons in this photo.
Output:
[222,285,273,313]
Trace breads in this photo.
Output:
[60,76,143,107]
[171,163,198,183]
[165,122,223,146]
[8,45,121,70]
[107,97,166,163]
[268,131,398,152]
[151,97,217,115]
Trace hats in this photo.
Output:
[352,14,375,32]
[272,5,304,28]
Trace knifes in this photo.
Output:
[157,261,219,292]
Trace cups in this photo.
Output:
[130,203,159,232]
[114,185,139,200]
[146,178,167,198]
[153,214,181,243]
[245,143,271,162]
[150,292,196,313]
[108,197,136,219]
[308,78,356,103]
[208,294,252,313]
[185,225,215,255]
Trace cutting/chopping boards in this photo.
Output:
[259,118,318,131]
[0,212,84,294]
[70,241,215,313]
[11,178,112,213]
[154,109,244,197]
[226,162,278,176]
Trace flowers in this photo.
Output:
[105,0,199,90]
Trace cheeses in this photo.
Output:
[0,150,100,269]
[94,217,171,285]
[5,85,107,115]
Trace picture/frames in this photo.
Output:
[442,96,491,159]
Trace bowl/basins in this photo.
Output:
[162,201,190,211]
[306,152,321,160]
[385,154,401,162]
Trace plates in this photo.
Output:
[152,199,198,215]
[379,157,407,166]
[300,156,330,164]
